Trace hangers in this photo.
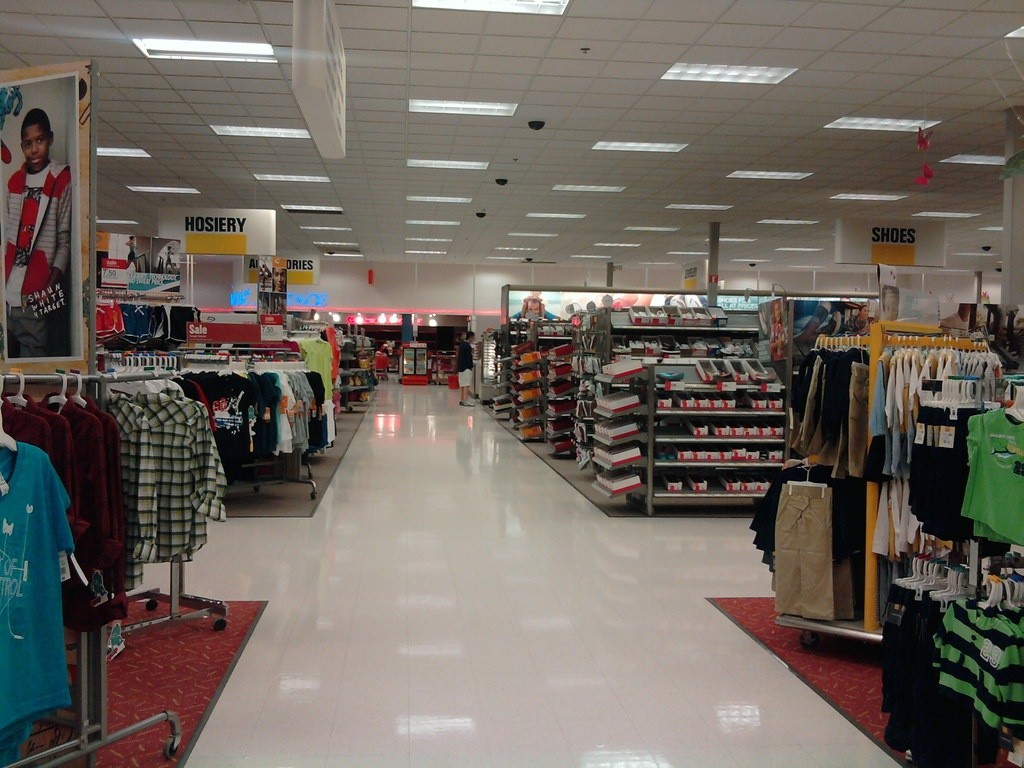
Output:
[0,318,332,768]
[812,333,1024,650]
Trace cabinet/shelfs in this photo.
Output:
[478,328,496,406]
[508,321,577,443]
[339,334,371,413]
[363,335,376,393]
[492,283,880,420]
[572,296,880,517]
[93,289,202,376]
[178,314,343,421]
[546,306,759,459]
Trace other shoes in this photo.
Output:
[938,311,969,330]
[610,302,789,493]
[460,401,474,406]
[548,314,605,470]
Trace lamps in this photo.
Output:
[823,116,941,134]
[407,98,518,118]
[411,0,570,17]
[132,38,278,63]
[659,62,799,84]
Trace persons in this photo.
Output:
[124,236,137,273]
[983,304,1019,337]
[458,331,475,407]
[793,301,845,345]
[383,347,389,373]
[844,301,874,333]
[939,304,978,331]
[259,258,272,288]
[272,267,287,293]
[882,284,900,321]
[3,106,73,359]
[509,295,560,320]
[249,259,255,268]
[164,246,174,273]
